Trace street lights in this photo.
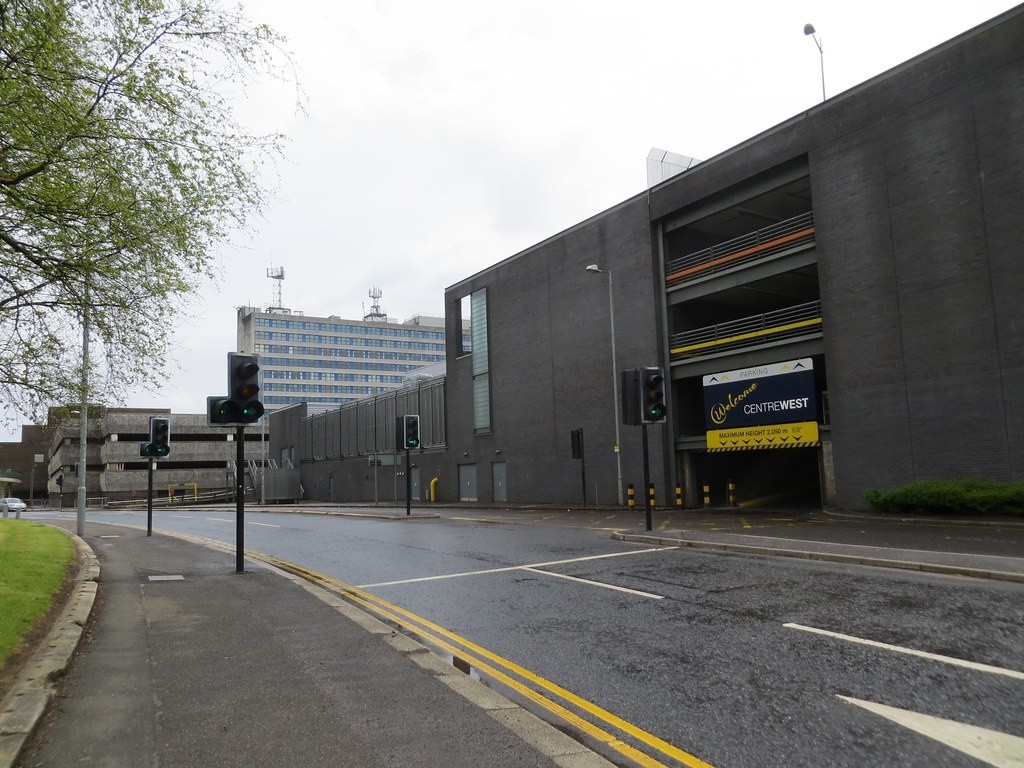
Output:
[804,24,825,100]
[586,264,623,506]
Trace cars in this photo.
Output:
[0,498,27,512]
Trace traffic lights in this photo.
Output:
[30,465,38,499]
[139,417,171,458]
[207,352,264,427]
[639,366,667,424]
[56,475,62,485]
[571,430,580,459]
[404,415,420,449]
